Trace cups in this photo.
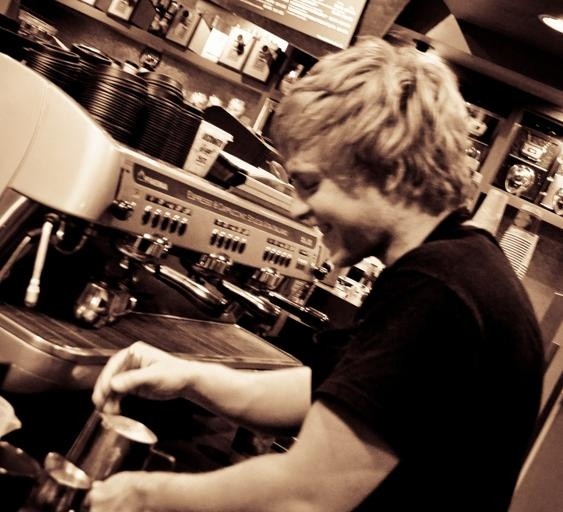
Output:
[39,450,91,512]
[79,412,175,484]
[1,442,39,507]
[181,120,235,180]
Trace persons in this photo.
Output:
[83,38,545,512]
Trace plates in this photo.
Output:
[18,12,202,169]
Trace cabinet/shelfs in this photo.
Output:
[350,0,562,236]
